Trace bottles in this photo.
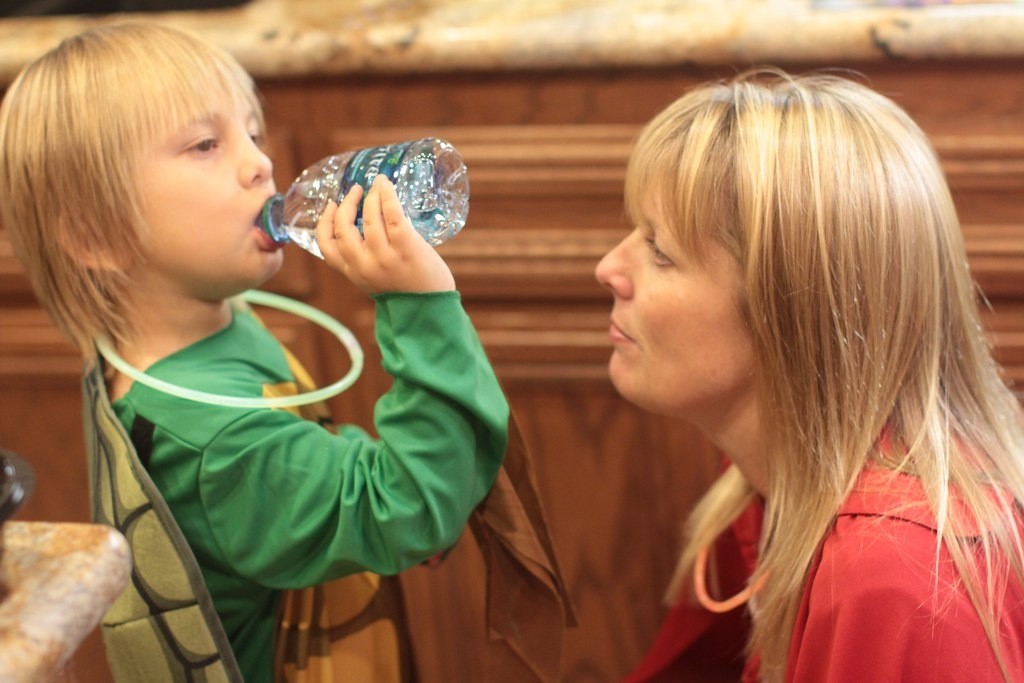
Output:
[255,136,470,256]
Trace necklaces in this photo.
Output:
[97,290,365,408]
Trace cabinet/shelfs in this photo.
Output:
[0,0,1024,683]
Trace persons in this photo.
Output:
[595,71,1024,683]
[2,23,509,682]
